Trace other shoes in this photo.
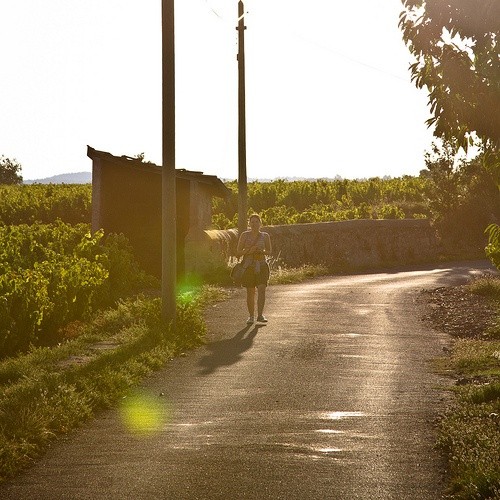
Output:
[246,318,254,324]
[257,316,267,321]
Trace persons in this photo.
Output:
[236,215,272,325]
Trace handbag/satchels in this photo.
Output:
[232,262,248,279]
